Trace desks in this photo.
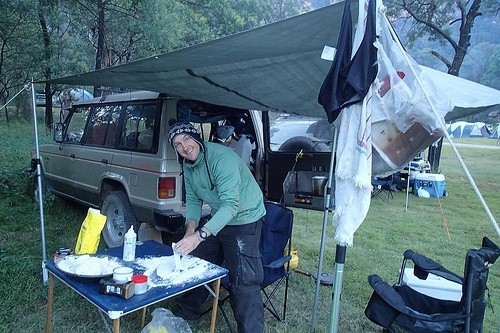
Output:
[42,239,230,333]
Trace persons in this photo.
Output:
[166,121,267,333]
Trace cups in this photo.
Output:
[312,175,328,196]
[113,267,134,281]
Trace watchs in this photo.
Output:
[196,228,208,240]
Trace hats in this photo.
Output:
[168,121,218,202]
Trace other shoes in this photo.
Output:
[172,294,225,324]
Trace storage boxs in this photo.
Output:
[398,267,463,302]
[413,173,444,198]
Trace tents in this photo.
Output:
[419,65,500,124]
[444,121,500,139]
[71,88,94,101]
[30,0,500,333]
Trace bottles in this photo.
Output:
[122,225,137,261]
[131,274,148,294]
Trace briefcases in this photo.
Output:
[413,173,445,198]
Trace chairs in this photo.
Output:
[364,237,500,333]
[371,174,395,202]
[200,201,294,333]
[94,119,117,146]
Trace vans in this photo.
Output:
[270,118,434,194]
[35,94,57,107]
[27,90,266,252]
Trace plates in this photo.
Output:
[54,254,127,278]
